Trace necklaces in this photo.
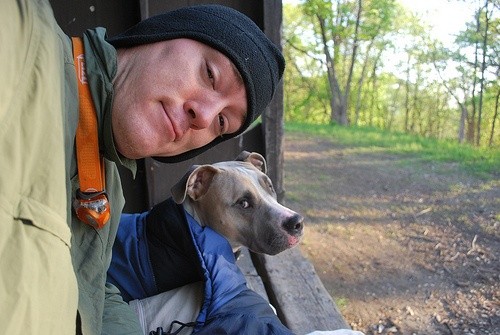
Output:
[72,36,111,229]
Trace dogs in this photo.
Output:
[170,151,304,261]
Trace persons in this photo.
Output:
[0,0,286,335]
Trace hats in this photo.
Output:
[102,5,285,163]
